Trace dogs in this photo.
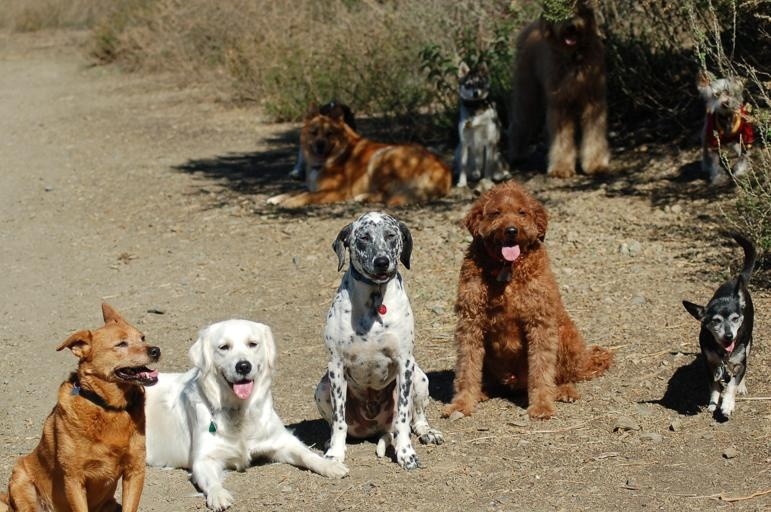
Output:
[682,230,756,419]
[697,77,749,187]
[441,181,615,420]
[507,0,611,179]
[144,320,350,511]
[0,303,159,512]
[266,102,452,210]
[315,211,444,471]
[454,62,513,187]
